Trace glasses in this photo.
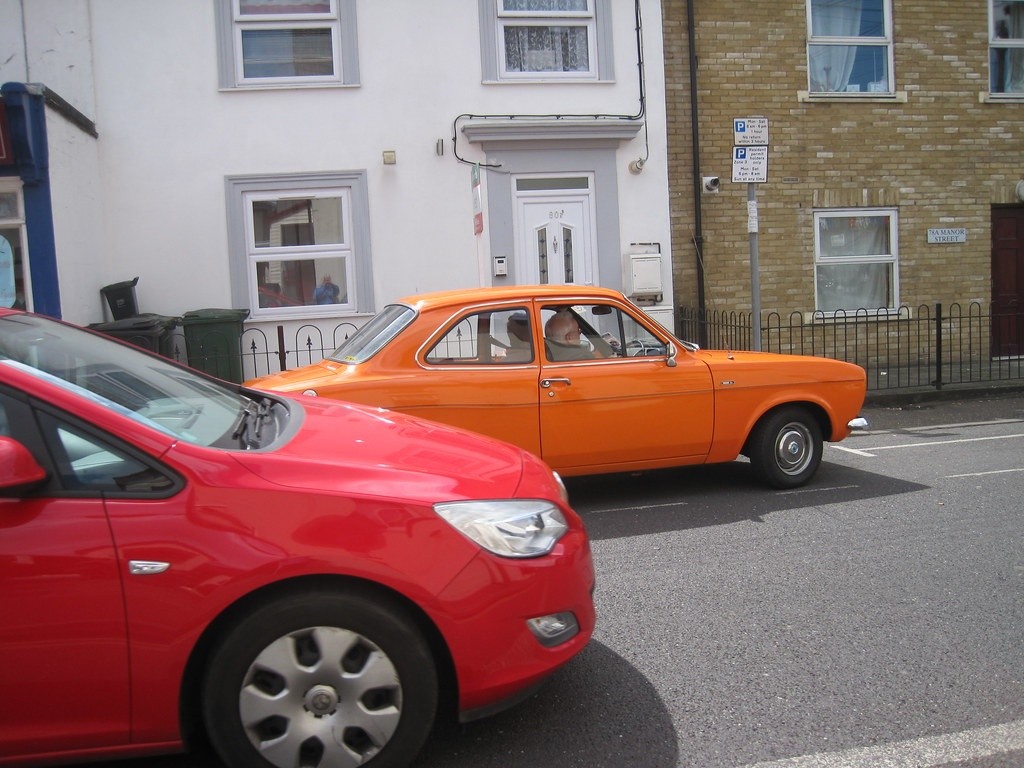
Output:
[577,328,583,335]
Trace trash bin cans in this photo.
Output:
[100,276,144,318]
[86,313,184,364]
[182,308,252,386]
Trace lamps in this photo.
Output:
[628,157,646,175]
[705,178,720,190]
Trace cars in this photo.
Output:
[241,284,868,492]
[0,308,596,767]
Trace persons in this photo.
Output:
[545,313,611,362]
[312,274,339,304]
